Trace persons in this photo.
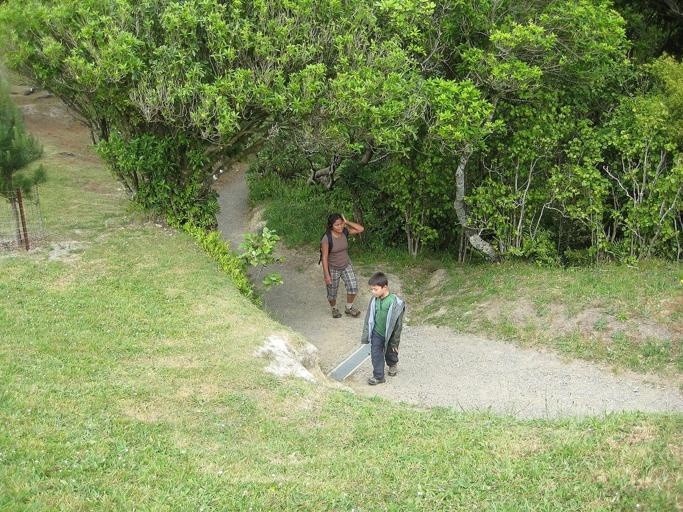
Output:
[320,213,365,318]
[361,272,405,385]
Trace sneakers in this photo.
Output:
[368,377,386,385]
[332,308,342,318]
[388,363,398,376]
[345,305,361,317]
